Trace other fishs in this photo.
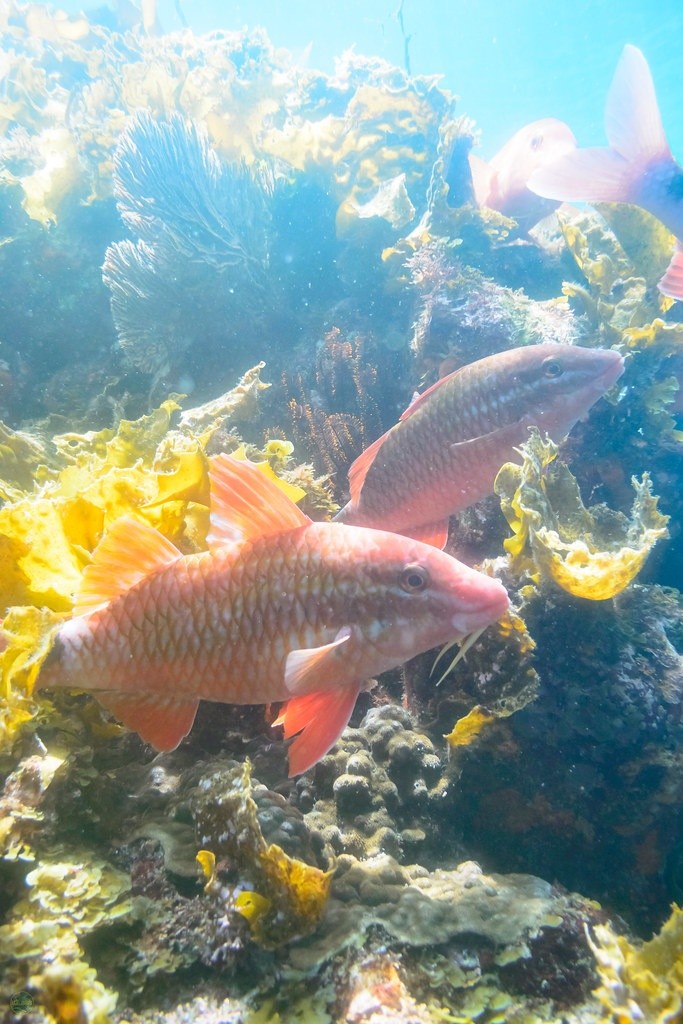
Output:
[328,344,625,550]
[2,452,508,777]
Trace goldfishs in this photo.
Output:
[518,45,683,252]
